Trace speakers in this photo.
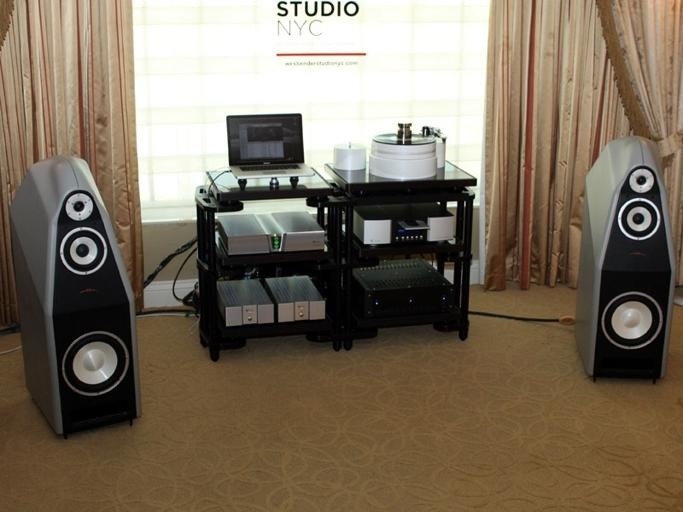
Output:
[575,136,675,385]
[9,155,142,440]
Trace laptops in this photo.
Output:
[227,113,315,180]
